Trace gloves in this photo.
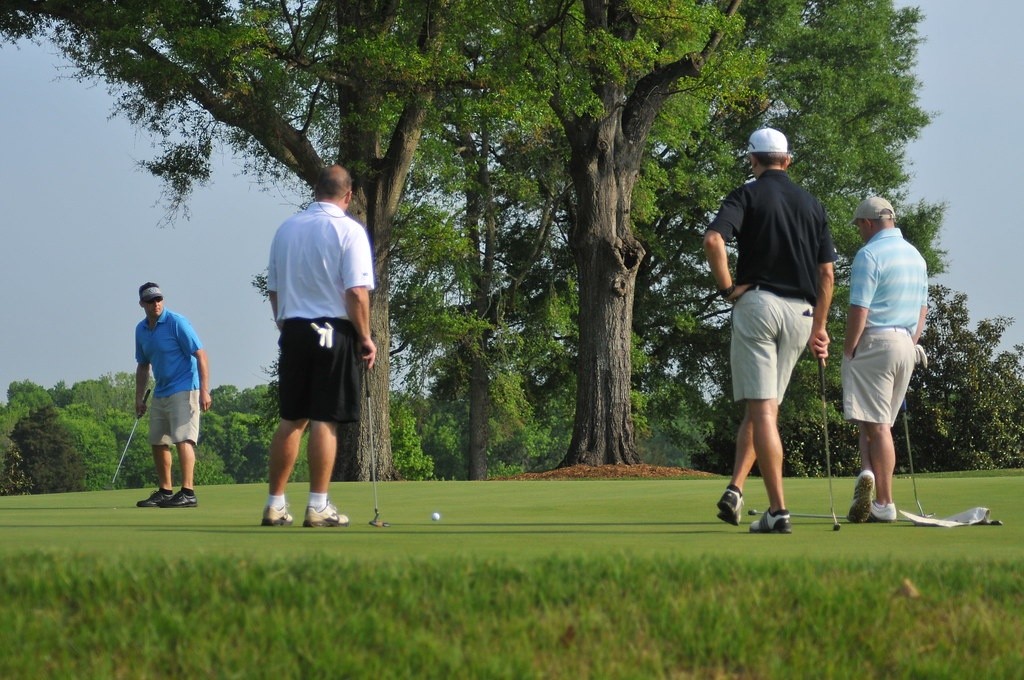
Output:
[311,322,333,349]
[914,344,928,370]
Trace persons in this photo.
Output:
[840,197,929,523]
[701,127,839,532]
[258,163,379,528]
[131,282,211,509]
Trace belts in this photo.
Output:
[866,326,911,334]
[745,283,816,306]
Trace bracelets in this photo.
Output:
[719,286,735,298]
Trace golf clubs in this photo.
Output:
[902,397,937,518]
[817,349,841,531]
[363,358,389,528]
[111,389,153,486]
[747,509,912,523]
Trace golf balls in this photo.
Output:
[432,513,440,521]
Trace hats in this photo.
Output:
[849,197,896,225]
[748,128,788,152]
[139,282,163,302]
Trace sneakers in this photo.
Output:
[261,503,293,527]
[136,490,175,507]
[157,490,198,508]
[716,484,744,527]
[847,469,898,522]
[749,505,793,533]
[302,500,349,527]
